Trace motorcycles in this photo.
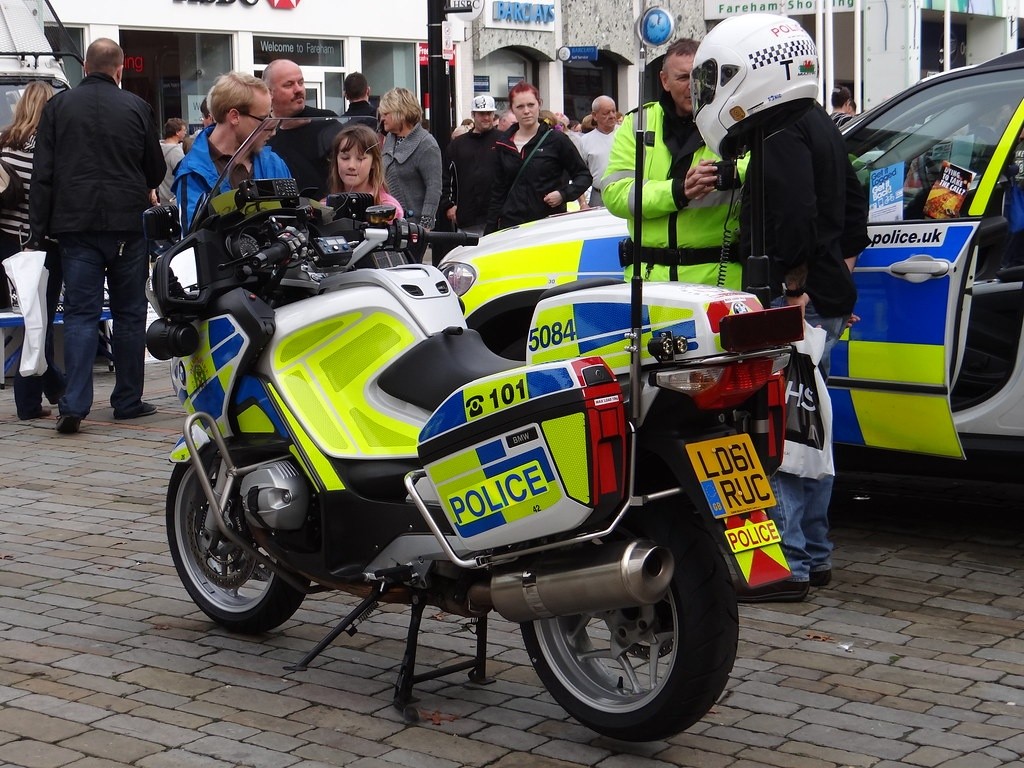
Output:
[143,117,808,743]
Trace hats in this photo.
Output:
[471,95,497,112]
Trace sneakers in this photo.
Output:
[114,403,159,418]
[55,407,84,433]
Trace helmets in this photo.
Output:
[690,14,819,160]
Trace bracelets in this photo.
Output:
[781,282,806,297]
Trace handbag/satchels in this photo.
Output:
[778,318,834,481]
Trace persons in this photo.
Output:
[846,99,859,116]
[600,35,753,292]
[149,57,624,260]
[29,38,167,432]
[0,81,61,421]
[688,11,871,602]
[829,86,855,128]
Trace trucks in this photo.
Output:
[1,0,85,132]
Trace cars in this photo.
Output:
[818,49,1024,505]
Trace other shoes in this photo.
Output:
[739,569,831,603]
[19,406,52,420]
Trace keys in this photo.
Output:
[118,238,129,257]
